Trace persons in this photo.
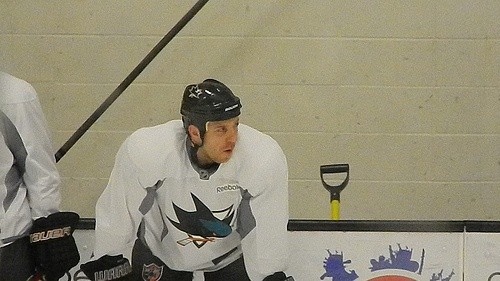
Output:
[78,80,292,281]
[0,70,82,281]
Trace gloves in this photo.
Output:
[29,211,80,281]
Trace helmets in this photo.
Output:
[180,79,242,147]
